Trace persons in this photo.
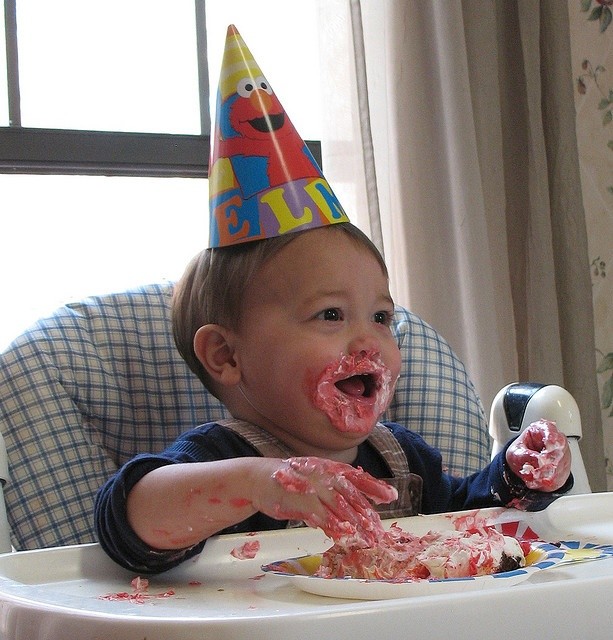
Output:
[94,222,574,576]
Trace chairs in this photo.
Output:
[0,282,491,549]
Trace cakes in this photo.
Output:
[322,520,527,578]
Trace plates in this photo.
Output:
[261,540,564,601]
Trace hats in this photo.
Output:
[209,24,350,248]
